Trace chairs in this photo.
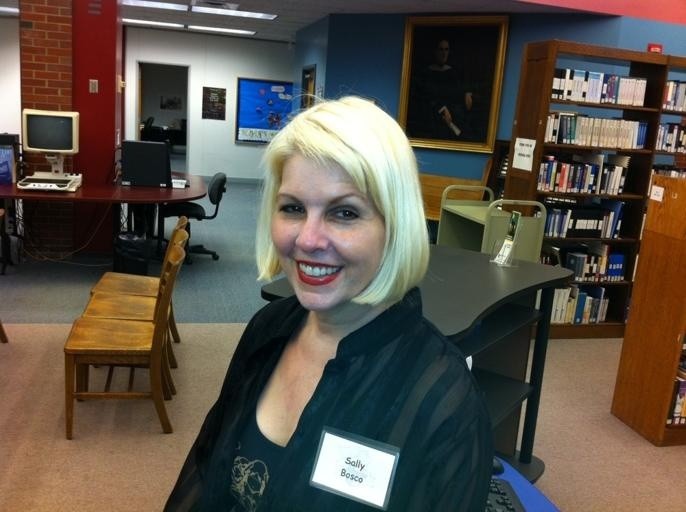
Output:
[63,215,189,441]
[160,172,227,264]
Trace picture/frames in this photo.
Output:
[397,15,510,156]
[235,75,300,147]
[300,62,316,109]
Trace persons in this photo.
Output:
[415,34,473,141]
[162,92,494,511]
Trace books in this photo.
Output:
[654,121,686,154]
[543,112,649,150]
[536,152,631,326]
[664,361,685,426]
[551,67,647,107]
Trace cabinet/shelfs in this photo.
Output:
[611,173,686,446]
[261,245,576,483]
[433,183,547,267]
[501,38,686,338]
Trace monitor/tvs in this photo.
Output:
[22,108,79,155]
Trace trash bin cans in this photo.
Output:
[112,232,151,275]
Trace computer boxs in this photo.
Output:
[121,139,172,189]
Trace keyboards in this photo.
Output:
[172,176,190,187]
